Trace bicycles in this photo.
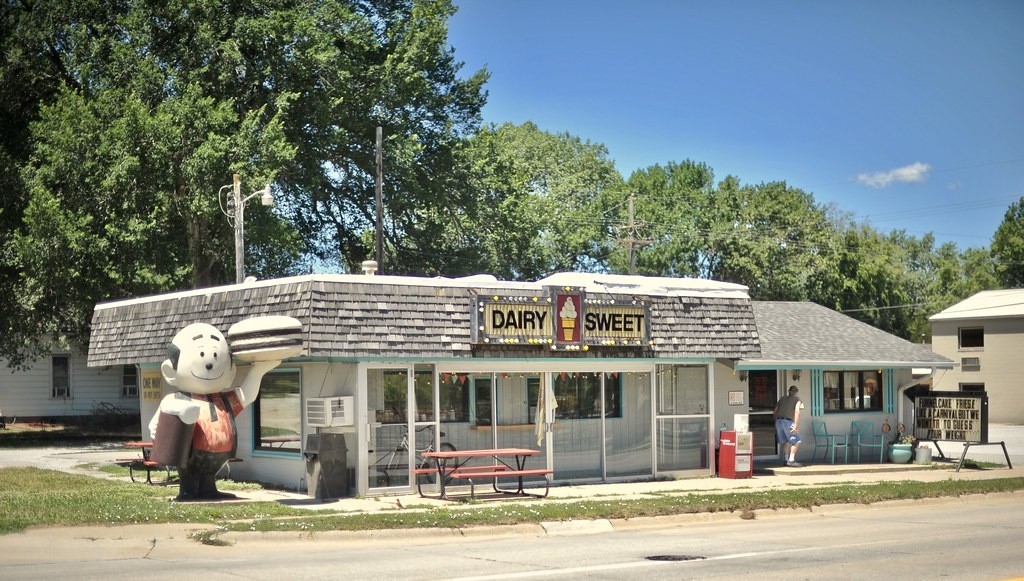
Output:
[380,407,459,486]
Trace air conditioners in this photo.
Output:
[306,395,355,427]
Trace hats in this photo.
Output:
[788,386,798,392]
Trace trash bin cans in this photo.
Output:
[303,434,348,498]
[718,429,754,479]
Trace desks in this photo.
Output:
[421,447,541,500]
[124,442,153,461]
[261,437,300,447]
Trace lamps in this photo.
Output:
[792,369,801,379]
[740,370,748,381]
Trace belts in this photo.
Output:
[777,417,791,421]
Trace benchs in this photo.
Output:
[450,469,554,501]
[116,461,172,484]
[412,464,507,497]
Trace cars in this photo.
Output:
[854,396,870,409]
[555,394,578,418]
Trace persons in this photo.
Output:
[774,386,804,467]
[149,323,282,501]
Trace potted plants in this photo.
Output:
[887,432,916,464]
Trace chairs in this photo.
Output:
[812,420,849,464]
[851,421,885,464]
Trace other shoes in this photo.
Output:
[784,459,801,467]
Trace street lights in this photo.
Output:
[233,173,276,283]
